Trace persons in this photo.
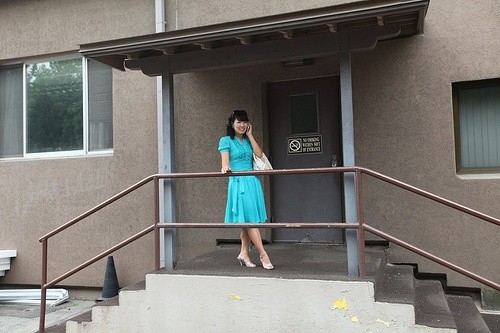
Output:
[218,110,275,270]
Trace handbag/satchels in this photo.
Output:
[253,152,272,170]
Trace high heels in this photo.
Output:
[237,255,257,267]
[260,255,274,269]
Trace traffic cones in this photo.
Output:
[94,255,120,302]
